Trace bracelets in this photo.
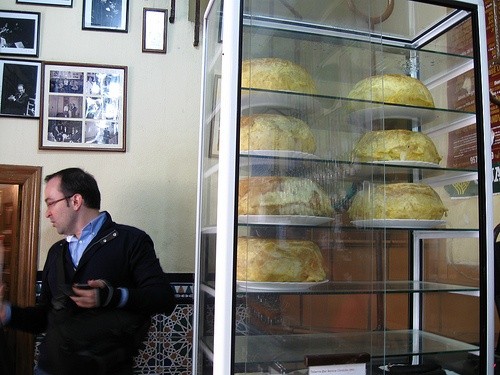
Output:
[117,287,128,306]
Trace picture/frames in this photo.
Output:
[142,7,168,54]
[0,58,43,119]
[0,9,41,57]
[82,0,129,33]
[39,61,127,152]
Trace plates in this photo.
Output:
[238,214,334,226]
[370,160,440,175]
[350,218,446,229]
[346,107,434,130]
[239,150,321,177]
[237,279,329,291]
[241,92,320,118]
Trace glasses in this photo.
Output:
[44,195,75,209]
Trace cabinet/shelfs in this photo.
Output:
[198,0,494,375]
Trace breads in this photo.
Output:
[238,56,446,283]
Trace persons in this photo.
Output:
[49,74,119,144]
[0,168,176,375]
[8,83,28,114]
